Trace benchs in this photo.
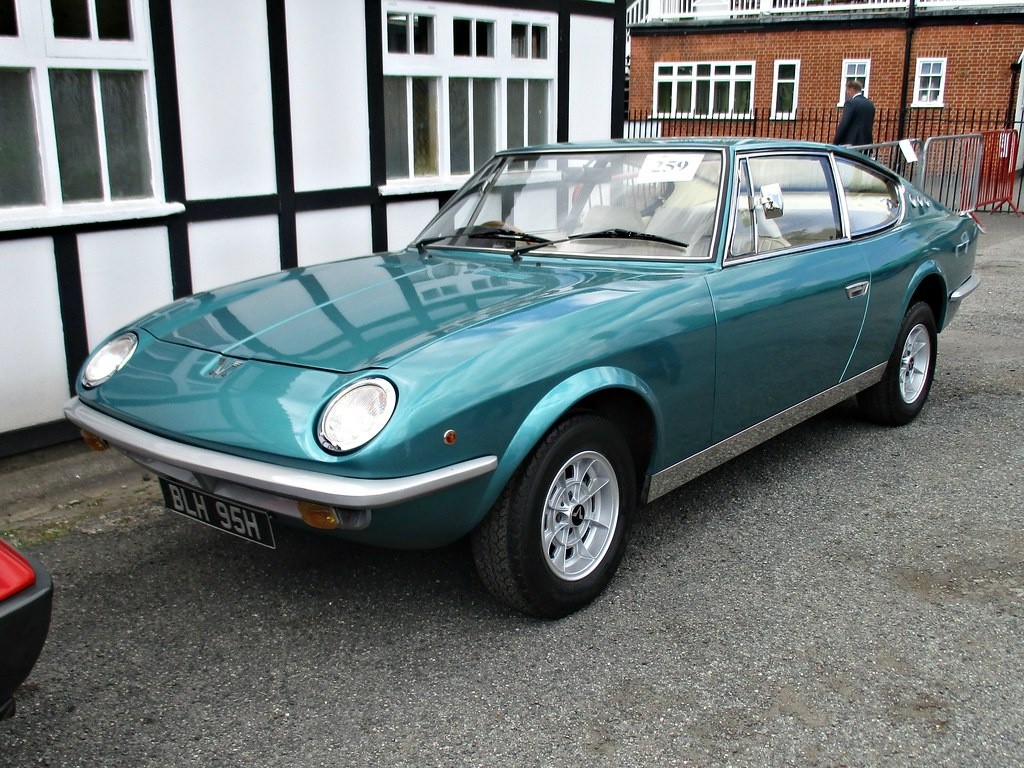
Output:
[646,200,888,248]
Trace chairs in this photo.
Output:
[582,205,646,235]
[692,207,791,258]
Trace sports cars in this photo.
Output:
[62,134,990,621]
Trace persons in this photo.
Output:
[833,80,875,156]
